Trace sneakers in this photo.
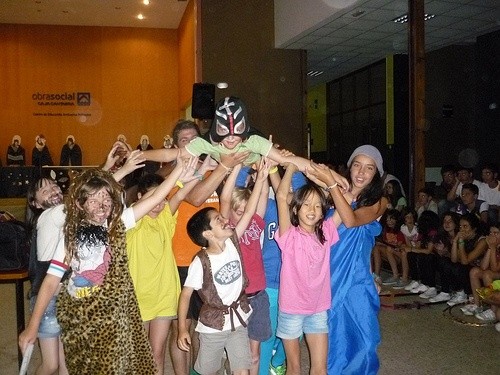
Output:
[447,291,465,306]
[461,304,483,315]
[429,291,450,302]
[405,280,421,290]
[495,322,500,332]
[410,283,429,293]
[392,281,410,290]
[419,286,437,299]
[475,309,496,321]
[382,277,399,285]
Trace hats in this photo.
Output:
[347,145,384,178]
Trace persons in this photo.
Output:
[6,98,500,375]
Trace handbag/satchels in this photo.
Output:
[0,211,30,272]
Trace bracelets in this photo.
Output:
[194,172,204,181]
[487,248,491,250]
[410,247,413,253]
[220,161,230,171]
[327,183,338,190]
[268,166,278,174]
[459,240,464,245]
[175,180,184,188]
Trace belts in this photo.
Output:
[245,290,261,298]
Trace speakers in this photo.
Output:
[191,83,216,120]
